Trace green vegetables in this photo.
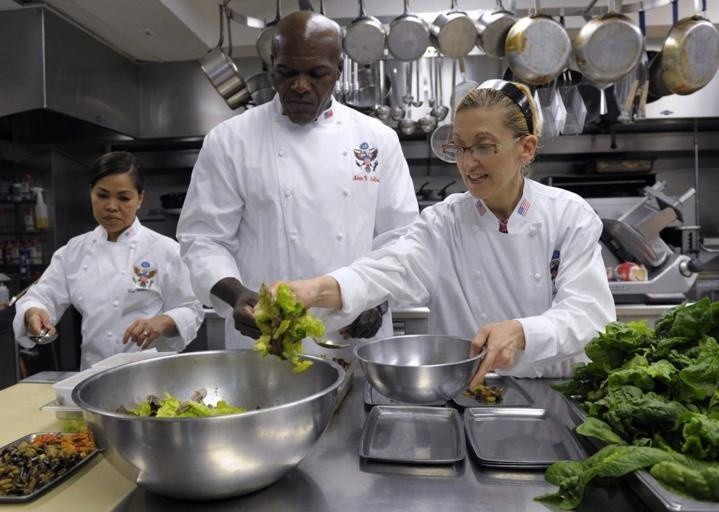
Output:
[532,296,719,509]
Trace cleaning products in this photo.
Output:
[29,186,50,229]
[0,283,10,310]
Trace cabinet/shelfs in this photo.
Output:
[1,150,57,371]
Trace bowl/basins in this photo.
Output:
[71,347,347,501]
[50,351,181,408]
[352,333,488,408]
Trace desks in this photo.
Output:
[0,370,139,511]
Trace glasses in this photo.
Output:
[442,134,529,157]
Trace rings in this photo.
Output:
[142,330,149,337]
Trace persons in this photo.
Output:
[11,152,205,375]
[180,9,420,366]
[253,79,620,390]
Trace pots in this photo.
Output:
[412,180,458,200]
[200,1,719,170]
